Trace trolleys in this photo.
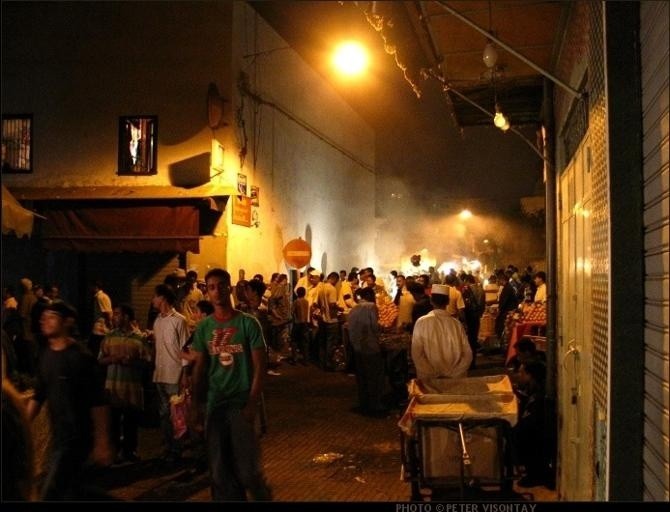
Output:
[403,375,521,499]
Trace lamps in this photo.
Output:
[481,0,500,68]
[491,69,506,129]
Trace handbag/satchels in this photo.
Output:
[169,367,192,440]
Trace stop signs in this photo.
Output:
[283,237,311,271]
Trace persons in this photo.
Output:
[2,251,549,502]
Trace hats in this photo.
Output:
[40,300,76,318]
[309,270,321,276]
[431,284,450,296]
